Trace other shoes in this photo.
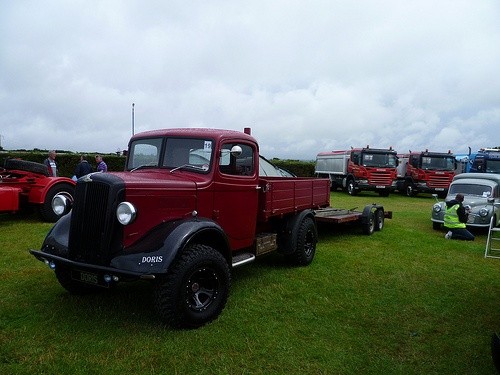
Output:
[445,230,451,239]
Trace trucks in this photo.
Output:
[454,145,500,176]
[396,148,458,199]
[314,145,400,197]
[28,127,332,330]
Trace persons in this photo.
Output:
[444,193,474,241]
[95,155,107,172]
[43,150,58,177]
[75,155,93,179]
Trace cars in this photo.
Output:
[0,157,77,224]
[430,173,500,232]
